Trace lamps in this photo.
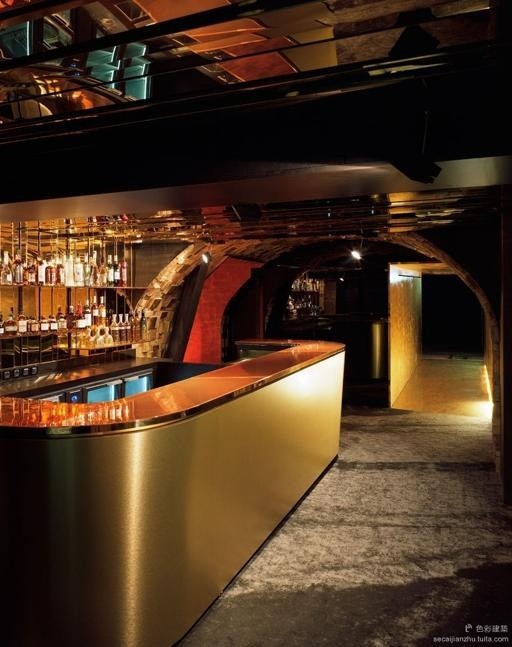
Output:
[347,240,366,259]
[201,240,219,265]
[338,271,348,282]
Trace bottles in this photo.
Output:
[0,250,146,348]
[0,397,134,428]
[288,278,318,316]
